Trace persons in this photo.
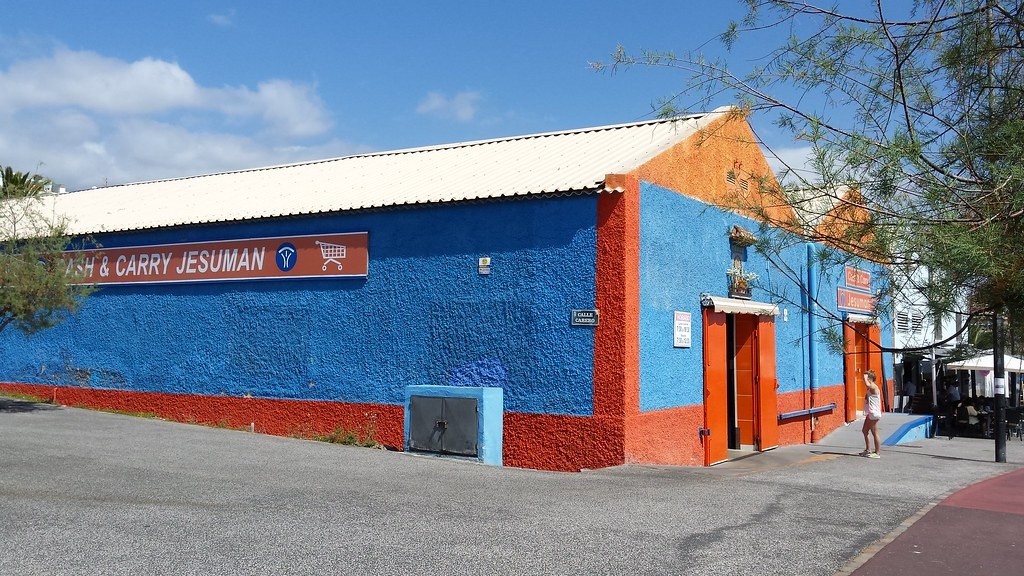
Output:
[859,369,882,459]
[903,377,916,409]
[944,378,989,439]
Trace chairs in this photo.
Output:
[954,396,1024,441]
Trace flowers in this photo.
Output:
[726,268,759,294]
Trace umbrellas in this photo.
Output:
[945,354,1024,373]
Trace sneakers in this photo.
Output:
[866,452,880,458]
[859,450,870,457]
